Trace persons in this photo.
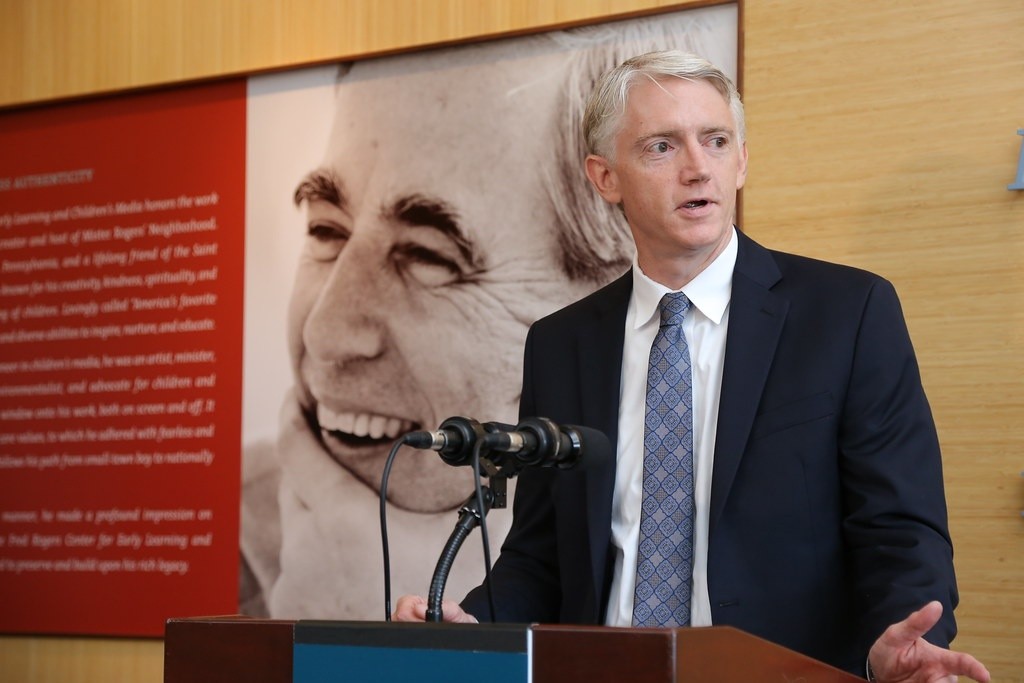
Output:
[237,14,730,622]
[390,50,990,682]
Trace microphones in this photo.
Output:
[402,415,611,473]
